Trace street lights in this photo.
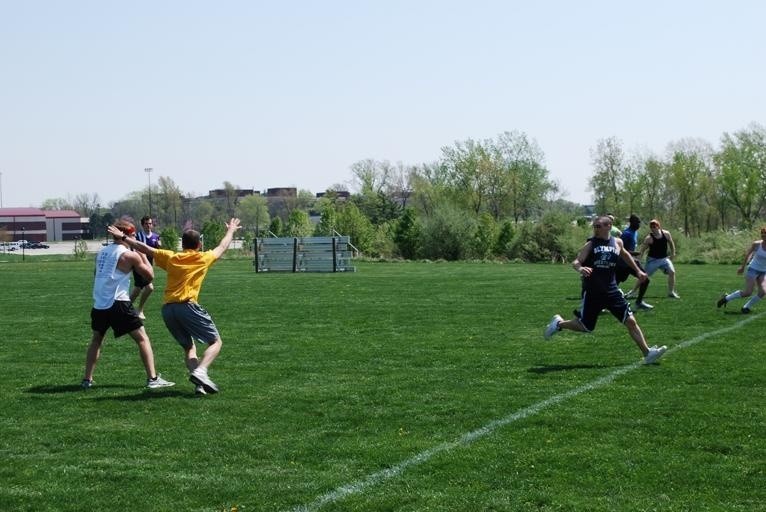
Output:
[20,226,24,261]
[144,168,153,218]
[104,222,109,247]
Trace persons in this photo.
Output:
[79,214,177,389]
[716,227,766,314]
[106,217,243,395]
[542,215,669,365]
[127,216,162,320]
[580,212,681,310]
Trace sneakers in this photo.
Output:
[83,379,95,387]
[147,376,175,388]
[669,291,680,298]
[634,301,654,309]
[645,346,666,363]
[544,314,563,337]
[189,369,218,395]
[717,295,727,307]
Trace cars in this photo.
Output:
[0,240,49,251]
[102,239,114,246]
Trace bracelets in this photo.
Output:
[121,233,128,242]
